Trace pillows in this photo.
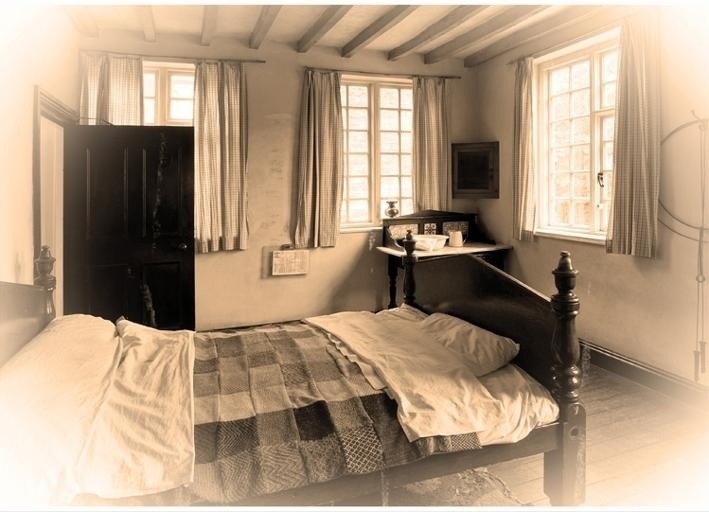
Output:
[419,312,521,379]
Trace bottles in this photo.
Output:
[384,200,399,218]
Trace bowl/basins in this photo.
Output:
[411,234,450,252]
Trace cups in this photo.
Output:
[447,230,468,247]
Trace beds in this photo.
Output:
[1,230,585,507]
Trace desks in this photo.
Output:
[376,243,513,309]
